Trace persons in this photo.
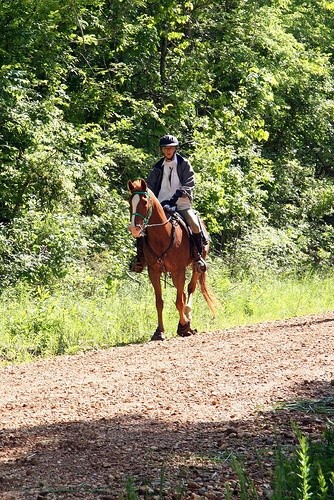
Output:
[129,135,208,273]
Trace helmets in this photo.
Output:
[159,135,179,147]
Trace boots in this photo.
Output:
[134,238,144,273]
[191,232,206,272]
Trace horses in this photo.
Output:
[127,178,217,342]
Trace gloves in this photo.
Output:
[169,190,182,207]
